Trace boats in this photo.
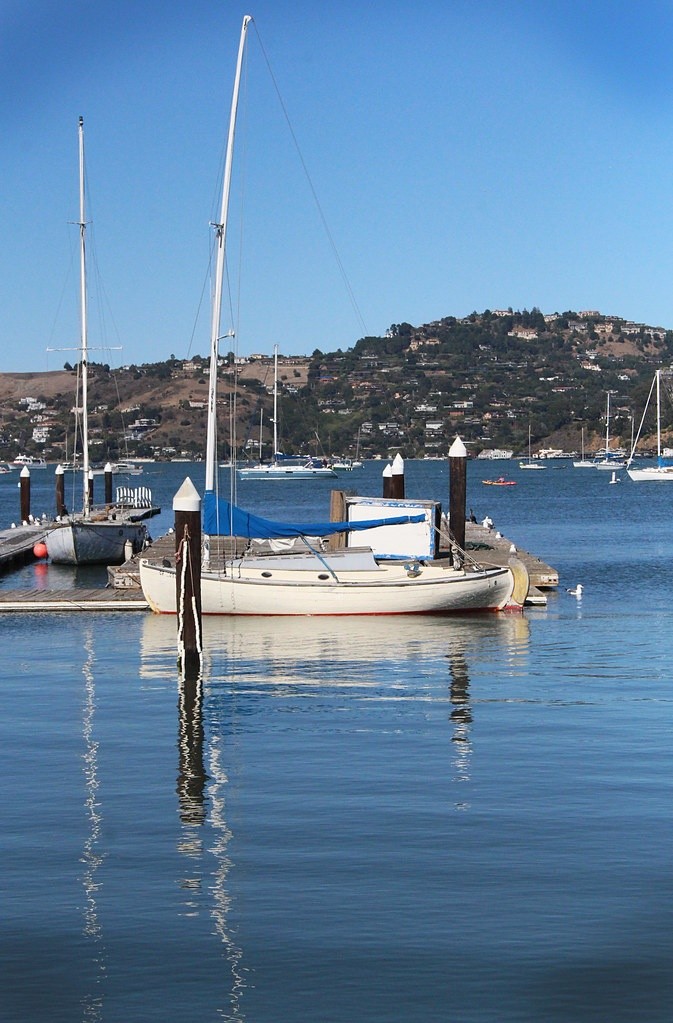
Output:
[572,427,596,468]
[235,343,339,482]
[42,115,150,566]
[481,480,516,486]
[626,369,673,482]
[7,454,48,470]
[517,425,548,470]
[90,462,145,475]
[595,392,625,471]
[326,458,353,471]
[139,12,527,617]
[60,431,85,472]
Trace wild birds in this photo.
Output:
[482,516,496,533]
[495,532,504,538]
[565,584,585,595]
[509,544,517,558]
